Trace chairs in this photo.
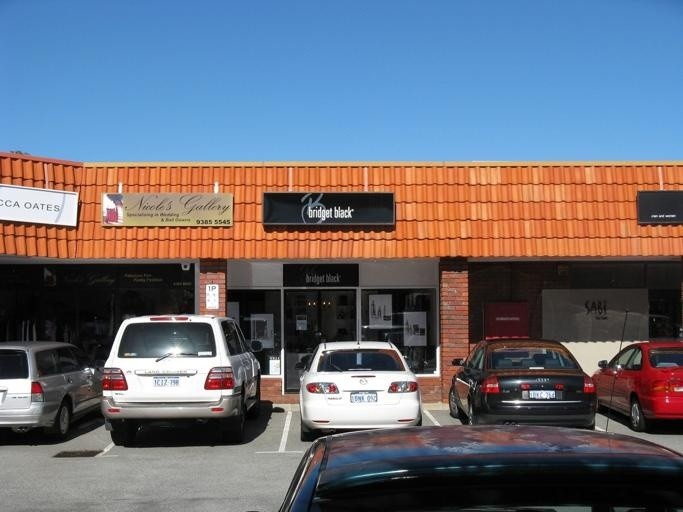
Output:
[533,354,547,367]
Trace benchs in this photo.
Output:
[498,358,560,368]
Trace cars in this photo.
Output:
[446,335,598,430]
[276,421,682,512]
[0,338,106,441]
[293,338,423,442]
[587,341,682,432]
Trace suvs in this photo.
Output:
[99,312,262,447]
